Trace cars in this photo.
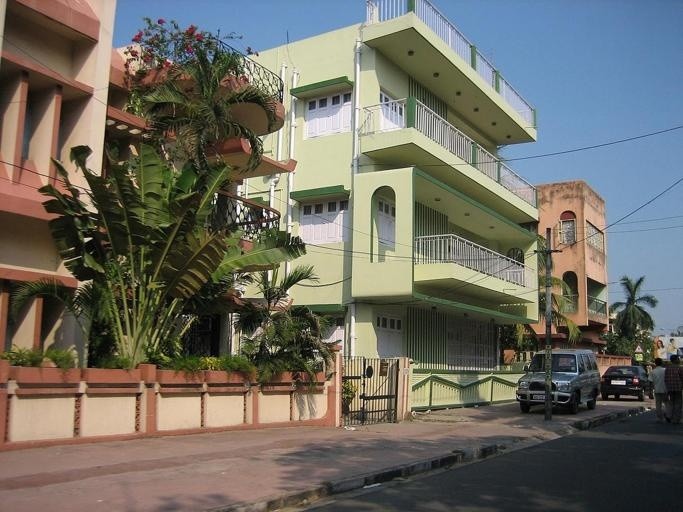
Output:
[601,366,654,400]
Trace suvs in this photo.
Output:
[515,348,601,414]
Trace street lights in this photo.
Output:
[660,327,675,337]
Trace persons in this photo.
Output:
[654,339,667,360]
[645,358,672,425]
[663,354,682,425]
[667,337,678,355]
